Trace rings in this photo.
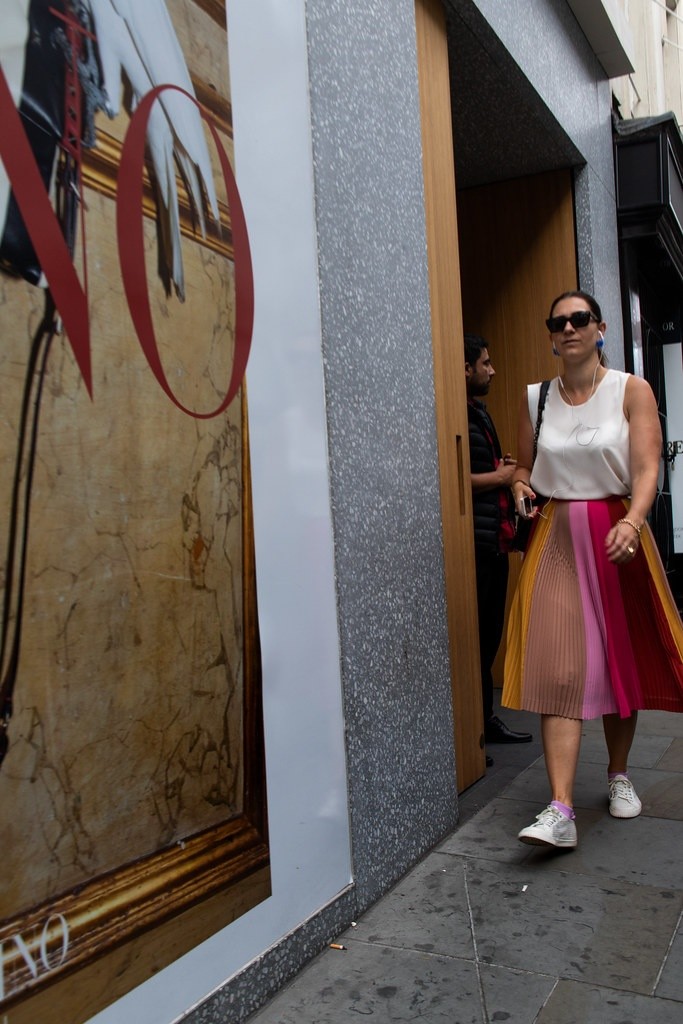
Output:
[627,547,634,555]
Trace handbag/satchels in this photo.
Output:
[514,381,551,551]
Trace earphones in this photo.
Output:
[552,340,556,348]
[598,330,604,340]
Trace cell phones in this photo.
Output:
[520,496,532,516]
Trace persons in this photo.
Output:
[493,290,683,847]
[464,335,533,743]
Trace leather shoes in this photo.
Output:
[486,756,493,768]
[484,715,532,744]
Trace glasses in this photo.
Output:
[545,311,598,334]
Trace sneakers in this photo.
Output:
[607,775,641,819]
[518,805,577,847]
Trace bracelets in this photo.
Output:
[618,518,640,538]
[512,480,528,488]
